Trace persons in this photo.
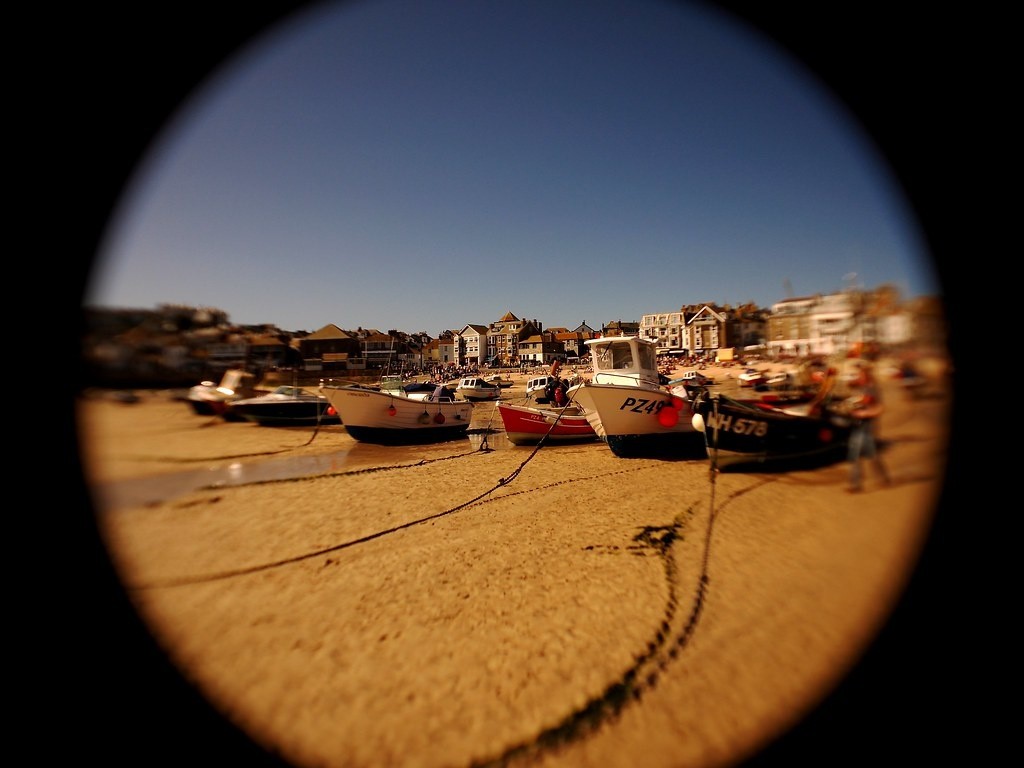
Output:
[832,346,891,493]
[655,343,811,422]
[378,358,594,409]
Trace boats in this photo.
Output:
[496,330,856,460]
[318,337,474,439]
[187,368,340,428]
[447,376,514,400]
